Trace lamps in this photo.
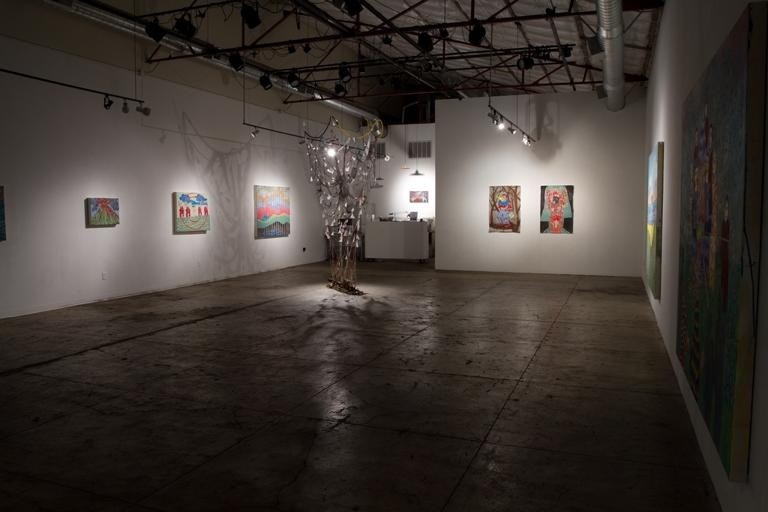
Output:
[234,0,262,29]
[507,122,517,135]
[99,91,151,115]
[416,22,576,69]
[145,9,198,44]
[248,125,261,138]
[229,32,391,97]
[335,0,365,16]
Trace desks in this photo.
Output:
[364,221,429,264]
